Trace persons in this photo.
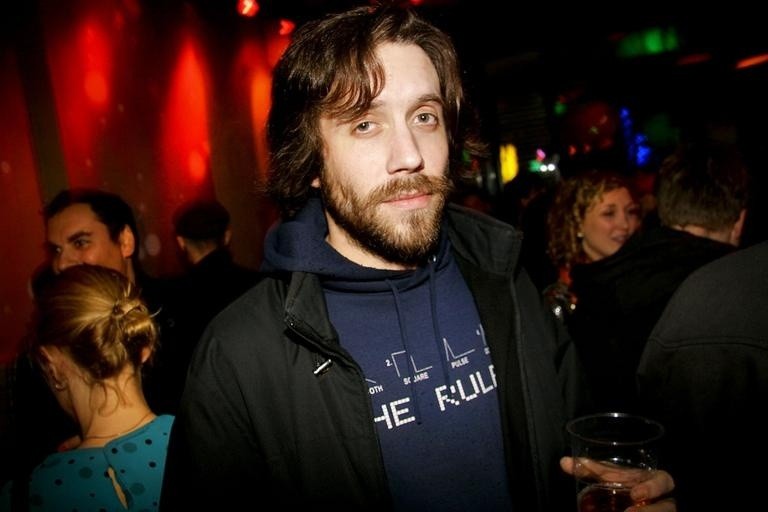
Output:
[158,4,681,511]
[3,144,765,509]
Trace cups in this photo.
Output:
[564,415,667,511]
[541,284,581,329]
